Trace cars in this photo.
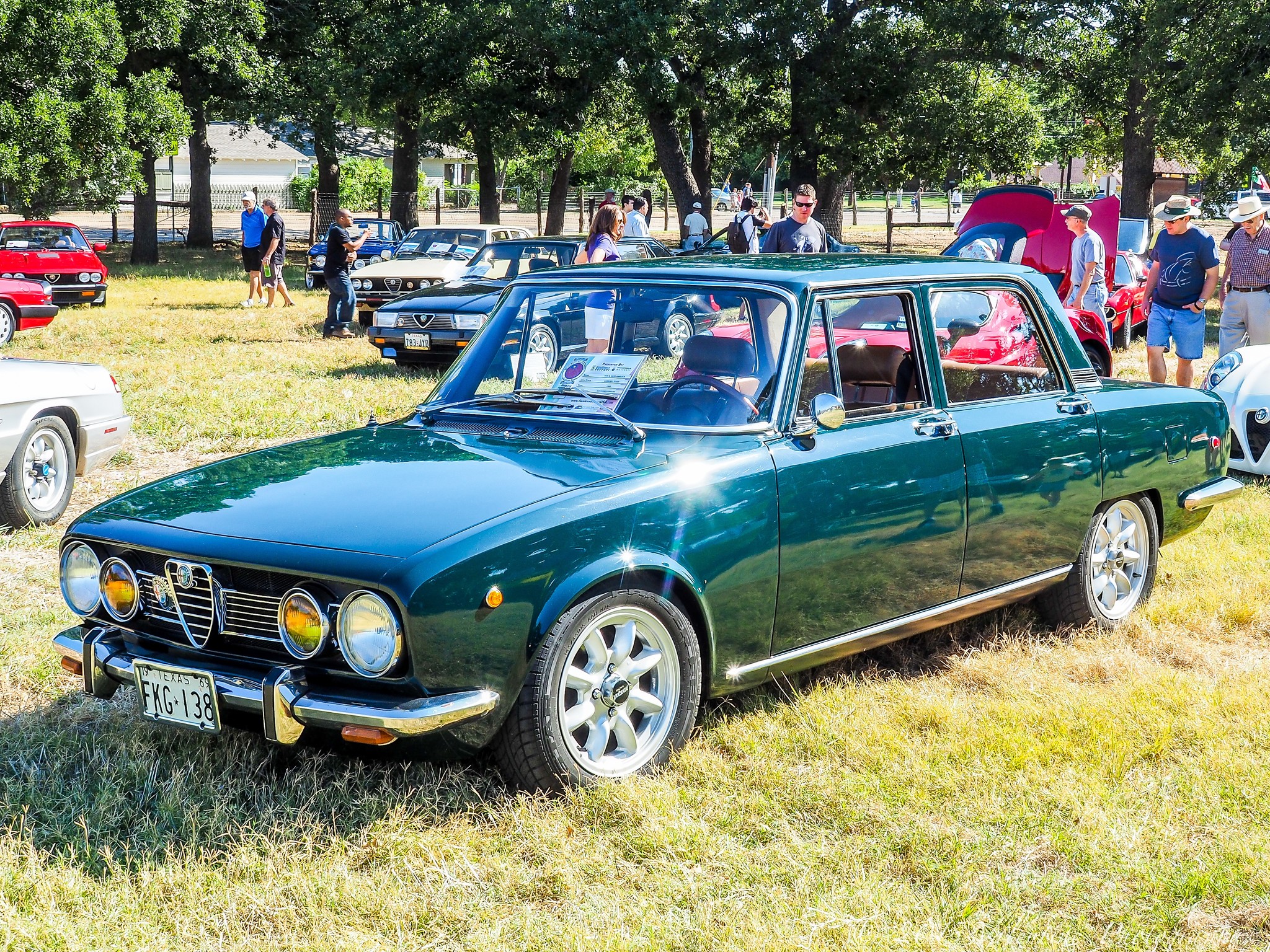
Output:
[0,220,134,530]
[51,247,1245,799]
[672,183,1269,479]
[306,217,719,380]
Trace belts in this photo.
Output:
[1074,280,1104,287]
[688,234,703,237]
[1229,282,1270,292]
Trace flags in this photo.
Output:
[1253,165,1269,193]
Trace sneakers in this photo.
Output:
[257,297,268,303]
[239,299,254,308]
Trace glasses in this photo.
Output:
[1164,217,1184,224]
[1066,217,1081,220]
[618,219,624,224]
[339,216,354,218]
[1241,216,1258,224]
[794,199,814,208]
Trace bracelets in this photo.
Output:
[1193,302,1205,312]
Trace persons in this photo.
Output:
[1218,206,1258,253]
[1214,195,1270,359]
[237,190,269,310]
[950,186,961,214]
[871,395,1229,531]
[256,196,295,310]
[1140,194,1221,387]
[1059,204,1111,354]
[321,209,373,341]
[909,195,918,212]
[573,166,862,364]
[915,187,923,213]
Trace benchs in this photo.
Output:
[907,356,1054,406]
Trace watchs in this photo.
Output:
[1197,297,1206,305]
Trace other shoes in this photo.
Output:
[739,316,748,321]
[282,303,296,307]
[322,332,333,339]
[331,329,356,338]
[956,211,960,213]
[264,304,275,308]
[952,211,956,214]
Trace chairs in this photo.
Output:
[638,333,759,427]
[835,344,906,411]
[4,235,26,246]
[529,258,556,272]
[52,235,73,248]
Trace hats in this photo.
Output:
[240,191,256,202]
[1153,194,1202,221]
[1060,205,1092,221]
[605,189,616,193]
[1228,196,1270,222]
[693,202,702,209]
[745,183,751,188]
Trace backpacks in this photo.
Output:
[723,184,729,192]
[727,214,755,254]
